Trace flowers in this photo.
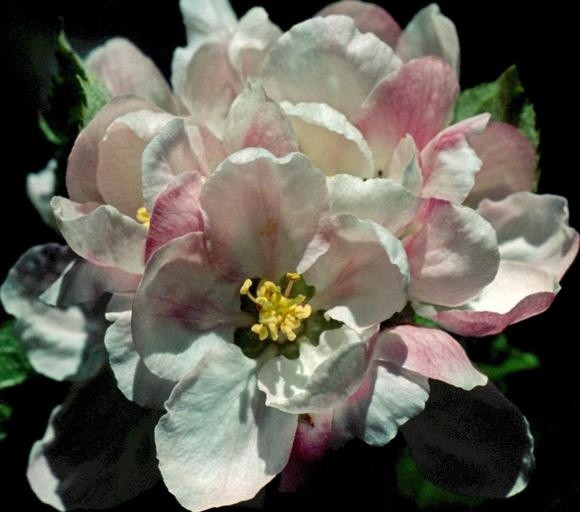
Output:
[0,1,579,512]
